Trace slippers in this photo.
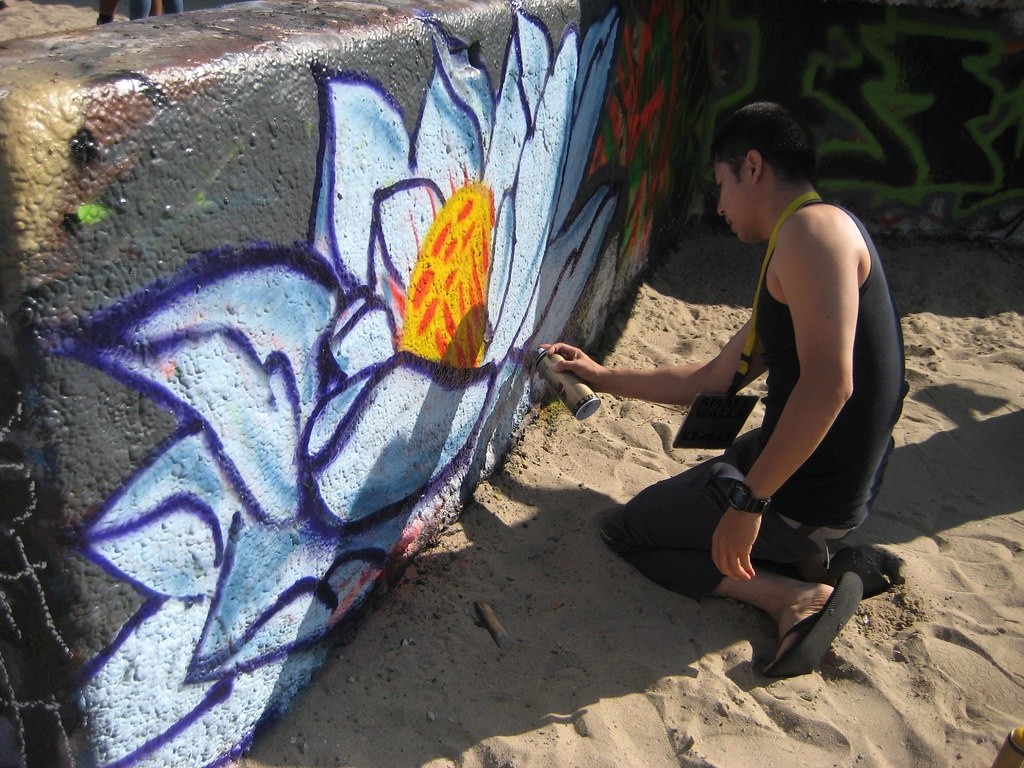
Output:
[824,546,892,598]
[763,571,863,679]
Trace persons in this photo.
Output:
[97,0,183,26]
[540,101,909,679]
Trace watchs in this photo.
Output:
[726,482,770,514]
